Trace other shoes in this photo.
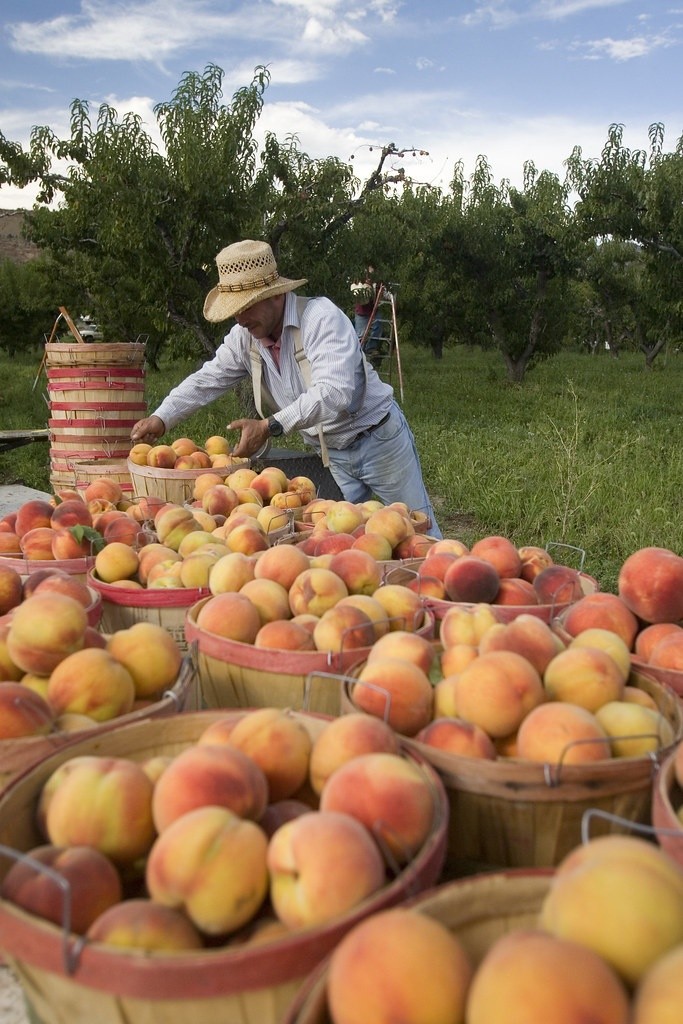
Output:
[371,350,382,355]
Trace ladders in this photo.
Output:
[359,280,404,405]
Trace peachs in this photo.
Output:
[0,432,683,1024]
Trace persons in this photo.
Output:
[353,266,397,367]
[130,239,445,542]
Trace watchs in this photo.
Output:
[267,416,284,438]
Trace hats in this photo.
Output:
[202,240,308,323]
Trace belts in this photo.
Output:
[350,412,391,444]
